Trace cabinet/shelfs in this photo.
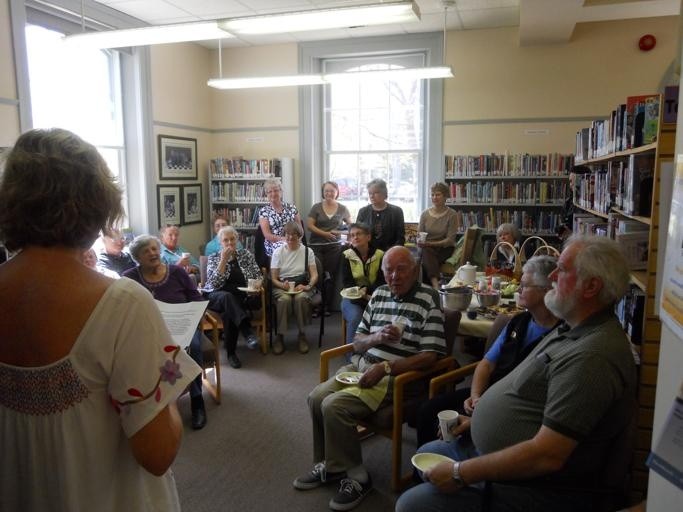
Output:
[445,174,572,256]
[208,157,295,243]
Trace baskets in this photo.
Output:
[490,236,560,284]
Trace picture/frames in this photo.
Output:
[183,183,203,225]
[158,134,198,180]
[157,184,183,230]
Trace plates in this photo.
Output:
[284,290,302,294]
[339,286,365,300]
[237,287,257,292]
[410,453,459,473]
[335,372,366,386]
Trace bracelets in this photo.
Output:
[381,358,390,373]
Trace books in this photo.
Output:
[208,156,279,251]
[443,153,566,253]
[564,92,661,352]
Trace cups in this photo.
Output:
[288,282,296,291]
[492,277,501,289]
[181,252,191,265]
[247,279,256,290]
[514,292,520,305]
[391,321,405,344]
[418,232,428,243]
[438,410,460,442]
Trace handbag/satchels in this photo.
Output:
[283,274,310,286]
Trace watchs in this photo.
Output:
[451,459,463,487]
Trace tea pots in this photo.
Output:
[456,261,478,281]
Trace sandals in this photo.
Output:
[325,309,331,316]
[311,309,320,318]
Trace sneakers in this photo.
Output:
[293,461,352,489]
[329,472,373,510]
[192,404,206,428]
[226,352,241,368]
[245,334,258,349]
[273,338,283,353]
[297,335,308,352]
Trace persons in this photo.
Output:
[354,178,406,285]
[306,181,352,318]
[415,182,460,288]
[202,226,264,370]
[156,225,202,289]
[416,253,562,450]
[0,127,196,510]
[392,232,642,511]
[258,178,304,257]
[290,246,448,511]
[269,219,318,355]
[489,222,534,273]
[96,226,137,277]
[79,248,120,280]
[338,222,388,364]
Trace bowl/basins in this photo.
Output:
[475,289,503,306]
[438,288,472,312]
